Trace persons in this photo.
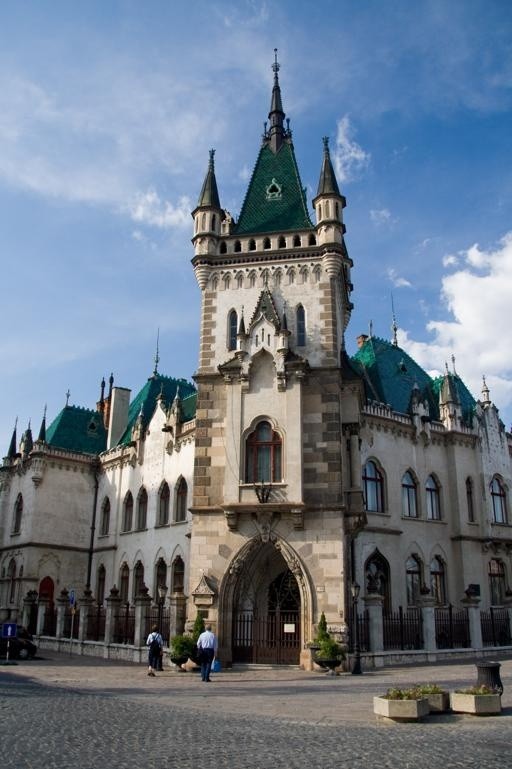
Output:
[196,624,218,682]
[153,655,164,672]
[145,624,163,677]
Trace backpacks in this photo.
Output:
[149,633,160,656]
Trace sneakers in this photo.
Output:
[202,677,211,682]
[148,670,157,676]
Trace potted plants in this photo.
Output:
[185,612,206,672]
[449,685,503,715]
[312,639,347,676]
[307,610,334,672]
[412,682,450,714]
[373,687,430,723]
[168,634,189,672]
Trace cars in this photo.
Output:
[0,625,36,659]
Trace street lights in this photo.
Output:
[156,585,167,671]
[352,581,361,674]
